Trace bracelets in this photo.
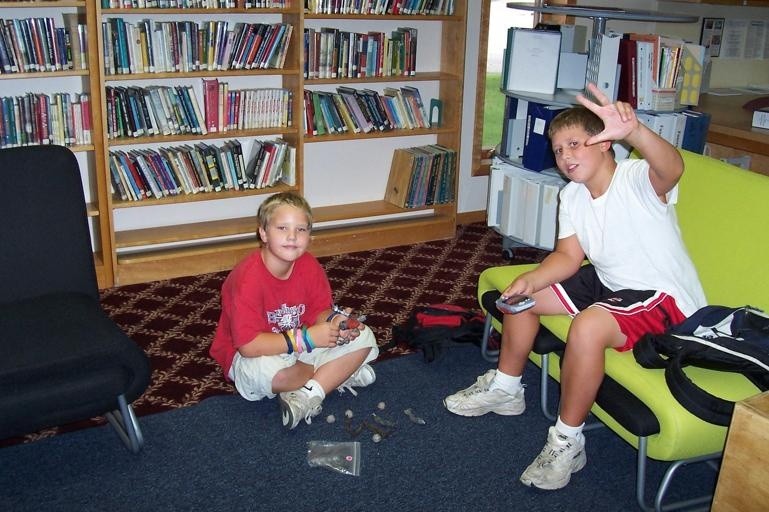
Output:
[280,325,315,355]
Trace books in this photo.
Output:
[303,26,418,80]
[0,93,92,149]
[102,17,294,76]
[637,111,712,155]
[384,143,458,209]
[514,176,535,240]
[620,32,707,109]
[106,78,295,140]
[102,0,294,9]
[109,138,297,201]
[486,164,504,226]
[540,183,566,249]
[303,85,433,138]
[522,177,544,246]
[0,17,88,74]
[303,0,455,16]
[500,173,520,237]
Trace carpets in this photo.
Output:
[1,341,769,511]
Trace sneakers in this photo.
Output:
[519,426,587,490]
[335,363,378,397]
[278,390,323,430]
[442,369,526,416]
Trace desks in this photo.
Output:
[690,87,769,177]
[475,143,769,511]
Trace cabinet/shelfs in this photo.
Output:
[499,3,699,248]
[1,1,113,288]
[96,0,468,287]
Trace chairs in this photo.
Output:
[2,144,149,455]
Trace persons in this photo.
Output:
[209,189,380,432]
[442,82,709,493]
[330,301,366,346]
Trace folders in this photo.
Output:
[654,112,710,156]
[488,164,558,250]
[590,30,622,103]
[524,105,556,172]
